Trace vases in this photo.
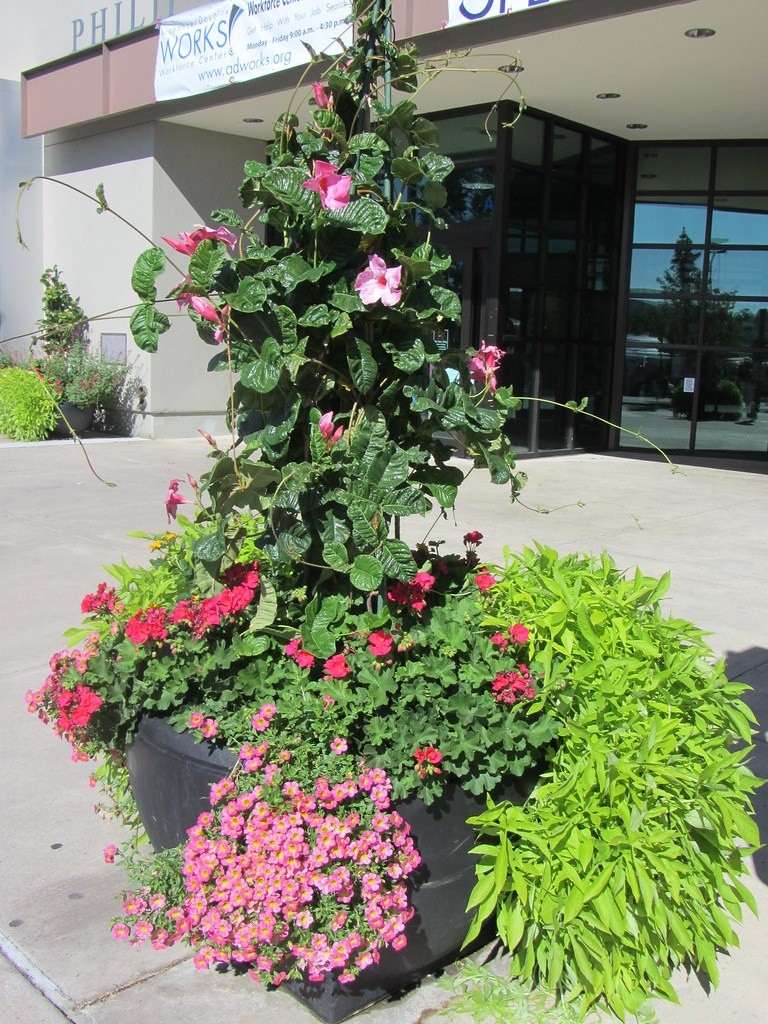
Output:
[126,712,537,1024]
[51,404,93,435]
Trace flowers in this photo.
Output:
[0,1,768,1024]
